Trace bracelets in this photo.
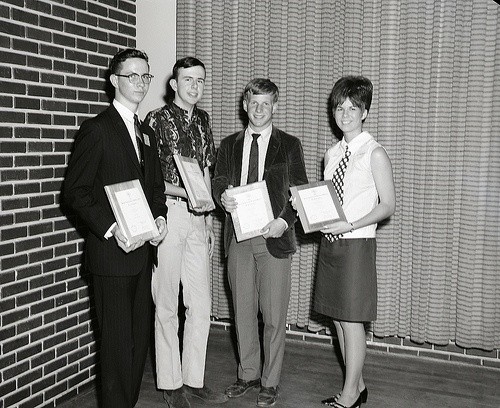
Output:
[350,223,354,232]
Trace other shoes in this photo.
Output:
[163,390,191,408]
[183,384,229,404]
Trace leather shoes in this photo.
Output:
[256,385,280,408]
[224,378,261,397]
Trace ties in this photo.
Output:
[323,146,349,243]
[132,113,144,167]
[246,133,261,183]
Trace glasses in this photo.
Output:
[114,72,153,84]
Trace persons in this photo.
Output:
[61,49,169,408]
[289,76,395,408]
[212,78,309,407]
[144,57,229,408]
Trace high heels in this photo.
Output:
[321,386,369,405]
[330,392,361,408]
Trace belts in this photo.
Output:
[165,194,187,202]
